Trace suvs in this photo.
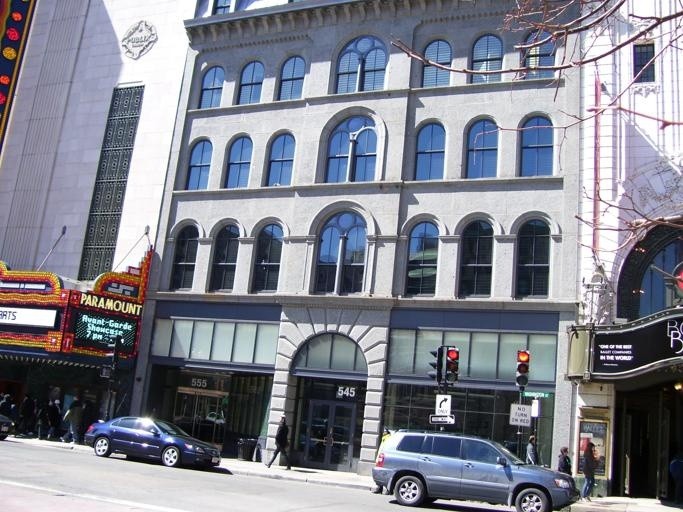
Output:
[372,428,580,512]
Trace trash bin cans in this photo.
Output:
[238,438,257,460]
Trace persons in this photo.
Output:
[0,389,92,444]
[524,436,539,465]
[670,451,682,503]
[556,446,572,475]
[582,441,596,500]
[264,416,292,470]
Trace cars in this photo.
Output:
[85,416,221,467]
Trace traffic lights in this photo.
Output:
[516,350,529,386]
[427,347,442,382]
[446,348,459,382]
